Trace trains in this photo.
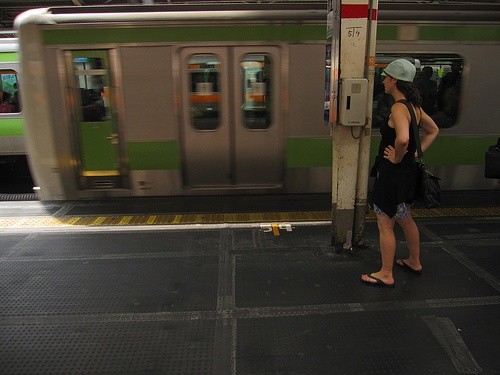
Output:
[0,30,29,156]
[13,0,500,202]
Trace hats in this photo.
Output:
[381,59,416,82]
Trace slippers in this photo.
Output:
[395,257,424,274]
[360,272,395,288]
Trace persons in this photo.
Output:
[251,70,269,116]
[438,62,462,120]
[199,63,216,90]
[361,58,438,288]
[0,91,15,113]
[0,83,20,113]
[81,88,104,121]
[372,67,454,128]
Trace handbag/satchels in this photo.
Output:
[408,161,446,210]
